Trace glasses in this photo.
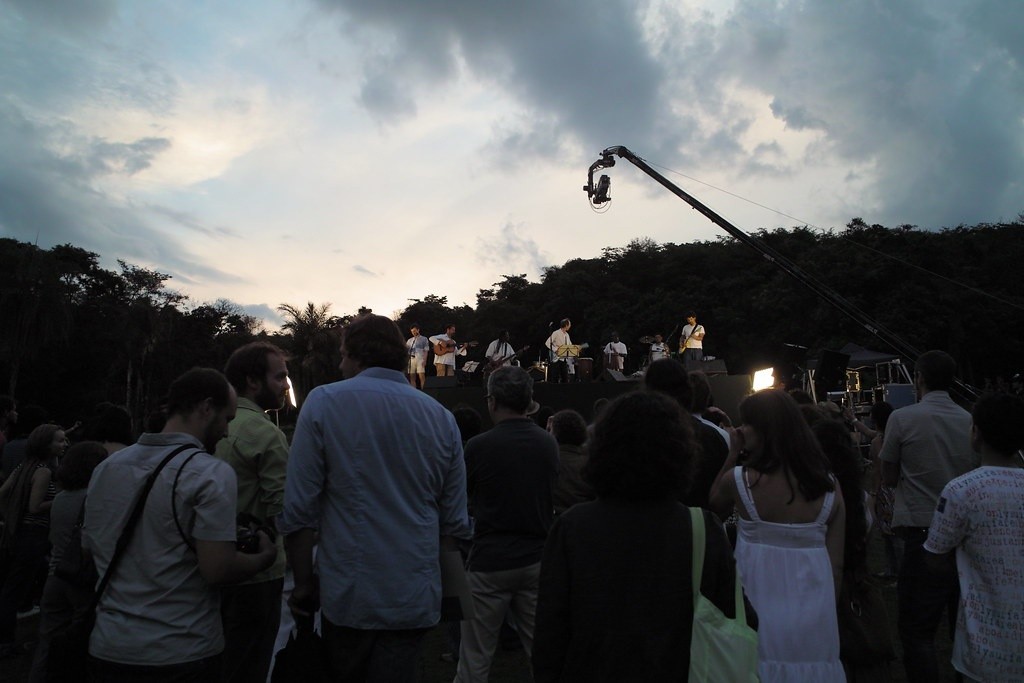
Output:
[483,394,492,401]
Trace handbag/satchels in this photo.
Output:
[38,530,97,652]
[689,505,759,683]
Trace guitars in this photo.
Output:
[433,338,479,356]
[486,345,531,372]
[552,343,589,363]
[679,326,703,354]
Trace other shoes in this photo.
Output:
[16,605,40,619]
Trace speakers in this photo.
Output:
[595,368,627,381]
[688,359,728,376]
[423,376,458,388]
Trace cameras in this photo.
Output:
[236,511,278,554]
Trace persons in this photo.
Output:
[603,331,628,372]
[81,366,278,683]
[428,323,468,376]
[530,390,759,683]
[0,313,865,683]
[279,314,474,683]
[841,348,1024,682]
[709,388,847,683]
[406,324,429,390]
[545,318,582,383]
[484,329,519,370]
[647,332,670,365]
[680,310,705,362]
[209,341,290,683]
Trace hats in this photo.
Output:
[1010,373,1023,381]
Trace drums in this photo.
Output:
[603,353,619,379]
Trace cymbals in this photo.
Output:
[640,336,655,344]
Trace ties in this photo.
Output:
[565,335,568,346]
